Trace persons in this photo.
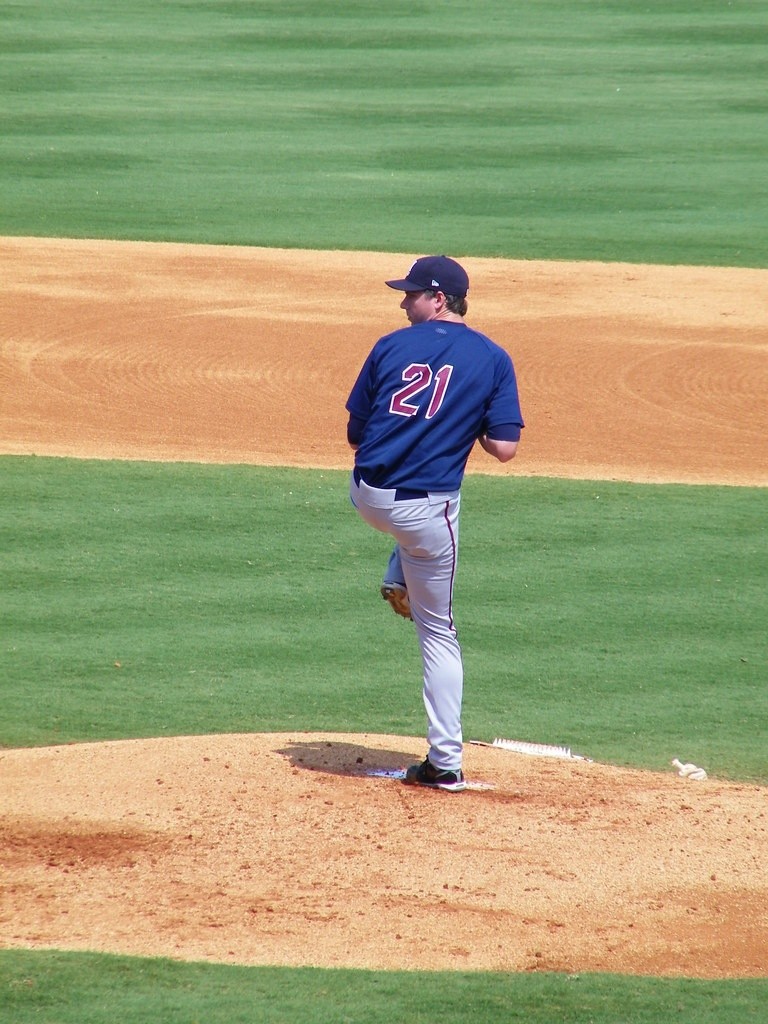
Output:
[346,254,526,794]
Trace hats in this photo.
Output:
[384,255,469,297]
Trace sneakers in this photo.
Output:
[380,581,413,618]
[406,755,466,791]
